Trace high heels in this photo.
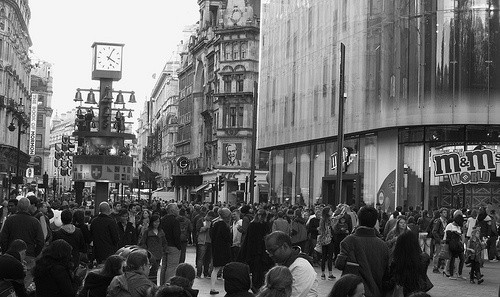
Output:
[442,270,451,277]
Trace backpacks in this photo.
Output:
[36,212,53,242]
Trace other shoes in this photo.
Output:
[433,269,440,274]
[195,275,201,278]
[328,275,336,280]
[207,275,211,279]
[210,291,219,294]
[449,276,457,280]
[458,274,466,280]
[217,274,222,280]
[489,259,498,263]
[320,275,325,280]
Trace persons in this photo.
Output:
[1,182,500,297]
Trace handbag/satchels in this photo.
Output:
[314,235,323,253]
[437,240,451,259]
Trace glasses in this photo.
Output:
[147,263,152,269]
[266,245,283,254]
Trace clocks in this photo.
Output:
[95,45,122,71]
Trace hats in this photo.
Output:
[454,214,464,223]
[217,208,231,218]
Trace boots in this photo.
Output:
[470,273,475,284]
[477,273,483,284]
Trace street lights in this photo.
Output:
[7,116,22,197]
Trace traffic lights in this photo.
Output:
[253,176,257,187]
[219,175,225,192]
[139,181,145,189]
